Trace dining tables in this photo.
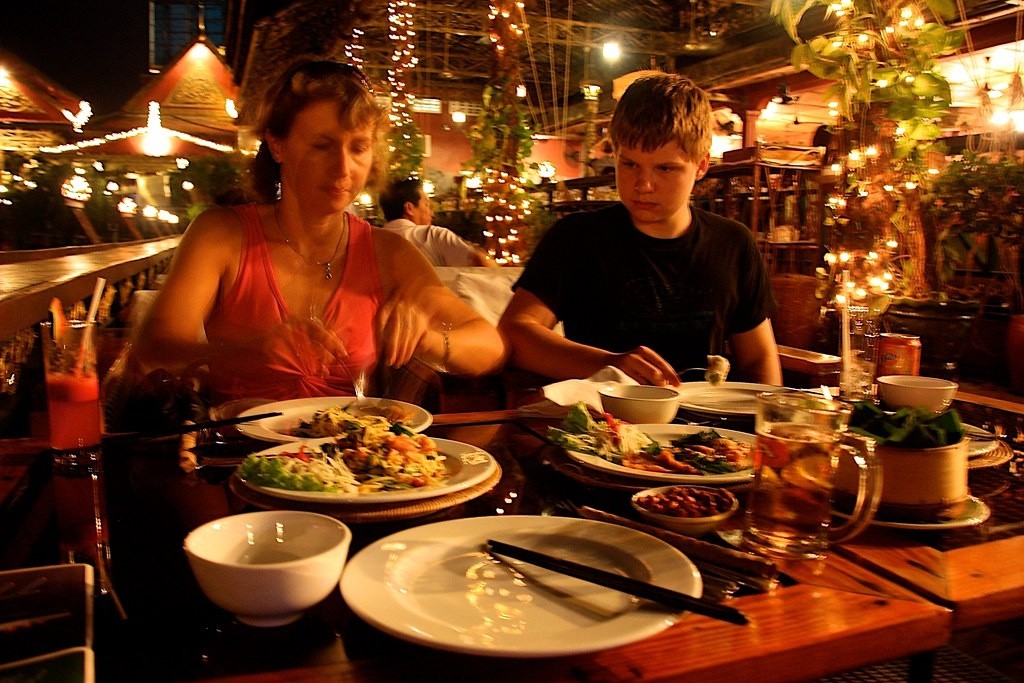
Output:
[0,378,1024,683]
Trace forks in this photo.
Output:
[676,367,707,378]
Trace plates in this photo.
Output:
[831,494,991,530]
[339,514,705,658]
[233,395,435,445]
[236,437,498,504]
[564,423,758,485]
[678,381,784,417]
[630,485,739,534]
[959,422,999,460]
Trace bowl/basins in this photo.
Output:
[182,509,352,627]
[597,385,683,424]
[876,375,960,416]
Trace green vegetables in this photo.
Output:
[237,456,325,491]
[546,402,738,473]
[847,401,966,449]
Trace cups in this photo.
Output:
[742,389,884,561]
[39,320,103,468]
[839,315,880,402]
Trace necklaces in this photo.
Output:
[274,201,346,281]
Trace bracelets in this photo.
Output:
[431,321,455,365]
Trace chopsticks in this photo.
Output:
[487,537,751,626]
[103,412,283,444]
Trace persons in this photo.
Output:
[378,176,499,271]
[132,54,516,440]
[495,76,782,391]
